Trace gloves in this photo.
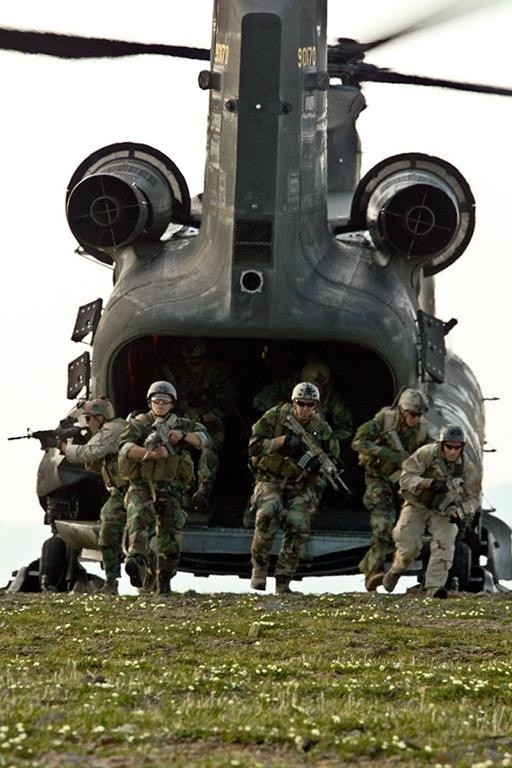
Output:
[377,445,404,469]
[281,433,306,460]
[44,433,63,450]
[448,510,462,524]
[428,478,450,495]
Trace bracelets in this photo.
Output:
[178,430,188,443]
[141,450,150,462]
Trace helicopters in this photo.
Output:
[0,0,510,598]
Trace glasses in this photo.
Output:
[85,414,101,424]
[403,409,423,418]
[443,442,463,450]
[294,397,316,407]
[149,396,173,405]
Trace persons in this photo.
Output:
[385,422,482,596]
[46,395,159,598]
[157,339,231,516]
[243,382,340,594]
[118,378,213,595]
[349,388,437,592]
[253,355,353,446]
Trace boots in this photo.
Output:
[190,476,215,515]
[275,573,294,594]
[382,566,403,592]
[138,569,157,594]
[431,586,448,599]
[94,579,119,595]
[364,568,385,590]
[250,568,268,590]
[157,568,177,595]
[242,499,257,529]
[124,552,148,588]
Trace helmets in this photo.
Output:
[146,380,178,402]
[397,387,429,416]
[81,398,116,421]
[290,381,321,403]
[299,357,332,386]
[439,424,467,444]
[180,338,217,359]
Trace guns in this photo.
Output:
[8,426,91,456]
[140,413,178,462]
[429,459,476,532]
[280,414,352,495]
[382,429,412,484]
